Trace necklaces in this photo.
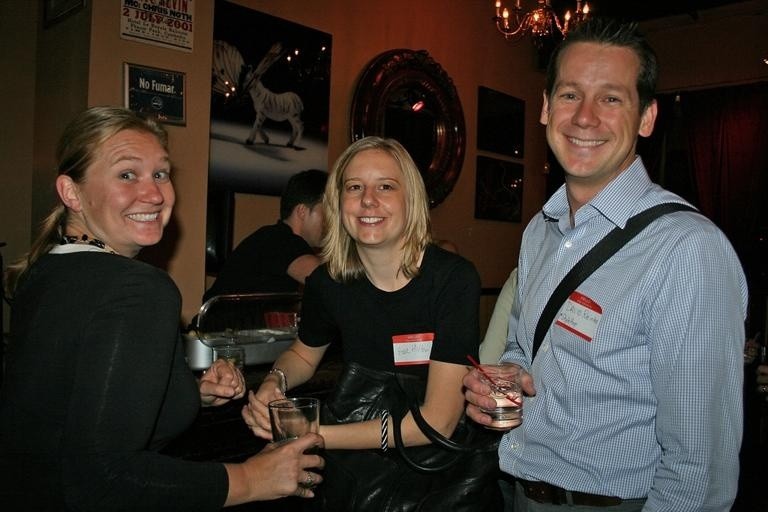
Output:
[55,230,118,256]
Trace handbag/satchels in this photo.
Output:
[320,361,499,512]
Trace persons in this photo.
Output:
[461,17,751,512]
[199,167,332,331]
[0,104,328,509]
[475,265,519,365]
[239,132,484,511]
[754,365,768,396]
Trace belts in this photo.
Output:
[522,479,621,507]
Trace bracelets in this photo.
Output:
[269,367,288,394]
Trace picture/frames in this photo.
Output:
[122,61,187,127]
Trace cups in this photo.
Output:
[268,397,322,491]
[212,346,247,376]
[476,362,523,429]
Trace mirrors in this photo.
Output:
[349,47,467,212]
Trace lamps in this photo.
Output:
[494,0,590,45]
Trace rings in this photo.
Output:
[762,385,768,393]
[304,471,315,488]
[297,488,308,497]
